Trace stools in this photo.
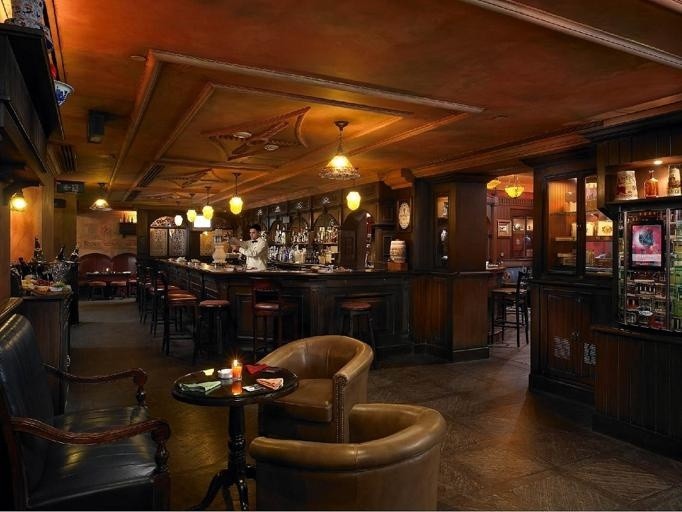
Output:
[190,299,233,366]
[87,281,107,300]
[111,281,127,299]
[337,300,380,372]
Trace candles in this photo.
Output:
[231,360,243,380]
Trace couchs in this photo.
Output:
[0,312,171,511]
[253,334,377,447]
[79,253,137,288]
[246,401,447,511]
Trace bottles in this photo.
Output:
[67,246,79,262]
[365,234,375,268]
[54,246,66,263]
[33,235,43,262]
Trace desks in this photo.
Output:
[87,271,132,301]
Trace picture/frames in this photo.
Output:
[496,218,513,239]
[396,196,413,233]
[629,220,666,271]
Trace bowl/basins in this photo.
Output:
[224,265,236,271]
[36,286,48,291]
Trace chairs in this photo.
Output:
[157,271,200,357]
[139,264,166,324]
[146,265,181,337]
[245,275,296,353]
[490,267,530,348]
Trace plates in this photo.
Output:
[168,259,174,261]
[50,286,63,292]
[200,268,208,270]
[175,260,187,262]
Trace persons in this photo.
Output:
[638,230,655,255]
[233,224,266,269]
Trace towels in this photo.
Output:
[179,380,221,393]
[201,368,215,376]
[243,364,269,375]
[256,378,284,390]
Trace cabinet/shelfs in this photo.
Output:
[568,109,682,463]
[309,189,342,267]
[251,206,268,231]
[119,221,137,237]
[0,22,71,205]
[266,201,286,263]
[20,283,75,416]
[286,196,310,263]
[41,261,81,323]
[521,145,617,413]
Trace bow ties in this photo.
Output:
[252,241,258,243]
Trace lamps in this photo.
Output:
[186,193,197,223]
[11,196,28,211]
[504,174,525,199]
[486,179,502,191]
[229,172,244,215]
[201,185,215,221]
[88,183,114,212]
[345,182,361,211]
[319,120,362,182]
[174,201,183,228]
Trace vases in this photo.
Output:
[52,79,76,106]
